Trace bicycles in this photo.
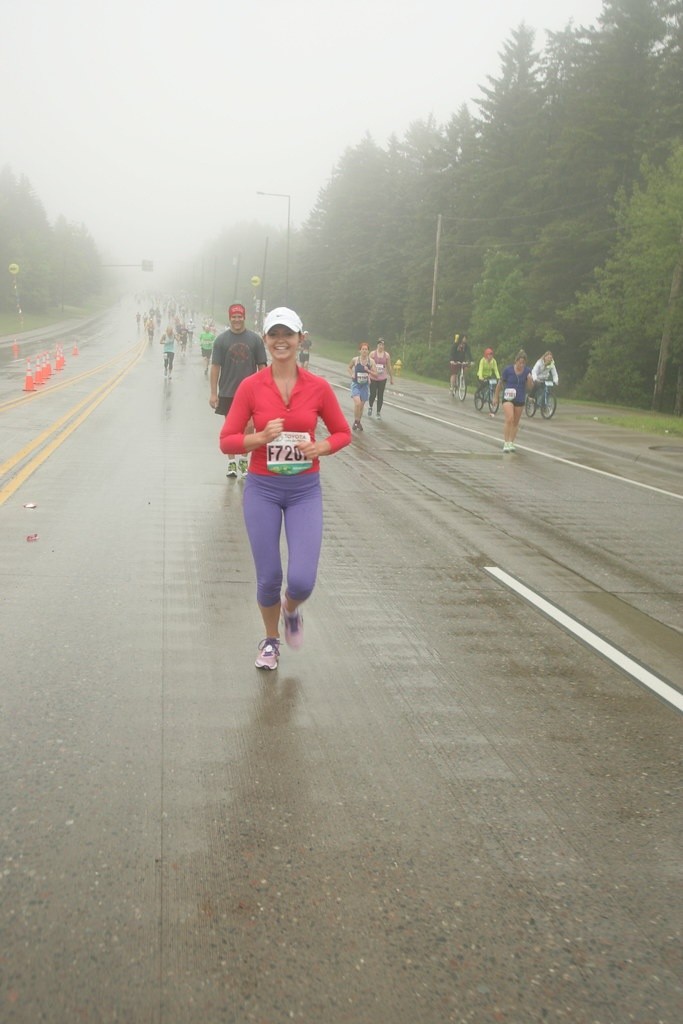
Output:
[526,380,557,419]
[452,362,470,400]
[474,377,501,414]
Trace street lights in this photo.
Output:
[256,191,291,310]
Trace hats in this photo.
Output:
[229,304,246,320]
[303,330,309,335]
[514,349,527,363]
[484,347,494,358]
[262,306,303,336]
[376,336,385,345]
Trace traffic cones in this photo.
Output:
[54,346,66,370]
[43,354,50,380]
[33,355,45,385]
[46,351,54,375]
[72,343,78,355]
[22,359,36,392]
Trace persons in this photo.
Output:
[220,306,352,672]
[475,348,501,399]
[300,330,312,370]
[160,326,180,379]
[368,338,393,420]
[208,303,268,479]
[133,295,217,380]
[347,342,378,432]
[493,349,534,452]
[447,334,474,396]
[529,351,559,401]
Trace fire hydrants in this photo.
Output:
[393,359,402,376]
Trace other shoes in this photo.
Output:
[503,441,509,452]
[352,420,358,429]
[238,456,248,479]
[368,407,372,416]
[509,441,516,451]
[226,459,238,477]
[357,423,363,430]
[255,636,282,670]
[281,597,303,649]
[377,414,381,420]
